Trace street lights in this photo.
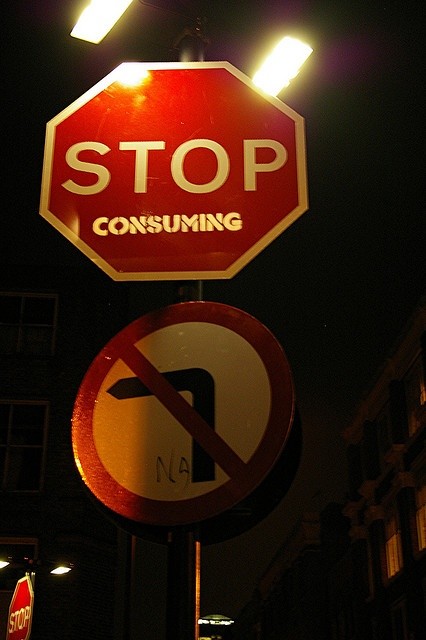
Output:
[68,0,317,638]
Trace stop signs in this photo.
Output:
[36,60,308,282]
[4,577,35,640]
